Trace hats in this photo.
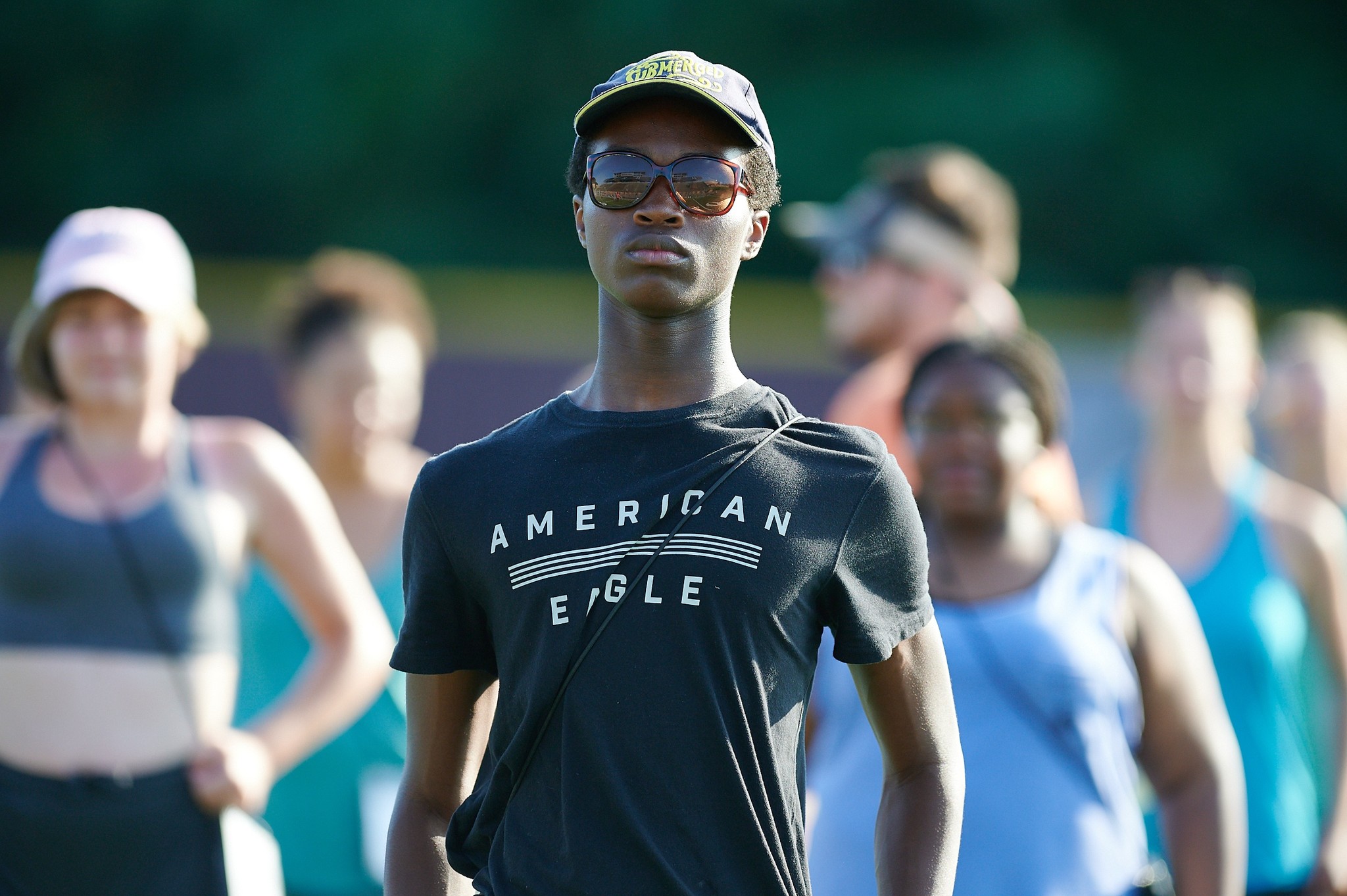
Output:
[36,205,198,323]
[572,50,777,181]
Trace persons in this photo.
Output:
[383,51,964,896]
[231,243,476,894]
[793,326,1245,895]
[783,139,1084,530]
[0,205,401,896]
[1261,312,1347,517]
[1080,273,1347,896]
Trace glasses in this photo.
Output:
[581,150,756,217]
